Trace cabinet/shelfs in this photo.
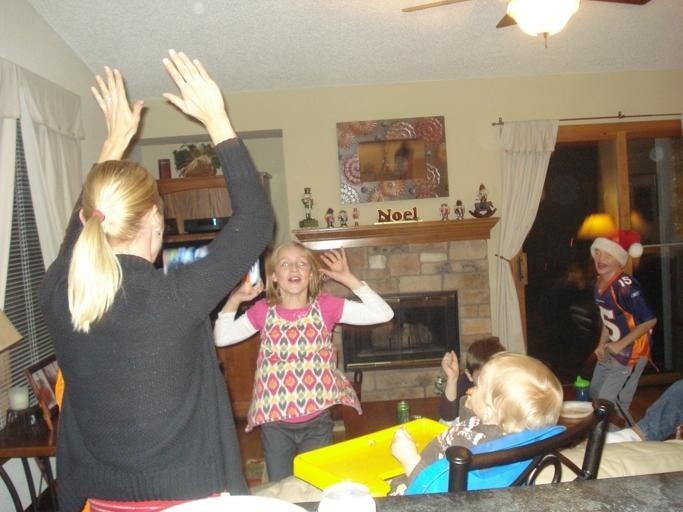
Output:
[155,172,273,418]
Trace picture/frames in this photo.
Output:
[24,354,65,432]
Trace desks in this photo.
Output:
[0,410,57,511]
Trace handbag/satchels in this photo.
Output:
[6,407,49,441]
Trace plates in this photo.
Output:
[559,400,594,419]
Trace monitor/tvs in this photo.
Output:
[156,239,266,306]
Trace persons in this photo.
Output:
[31,46,277,512]
[438,204,451,221]
[433,335,510,425]
[453,198,466,220]
[337,209,350,227]
[582,229,659,431]
[468,184,497,219]
[604,377,683,444]
[324,207,336,228]
[301,188,314,220]
[352,207,360,227]
[212,240,398,490]
[384,350,564,498]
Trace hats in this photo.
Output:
[591,229,643,269]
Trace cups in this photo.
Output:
[575,375,590,401]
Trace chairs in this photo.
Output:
[446,399,613,494]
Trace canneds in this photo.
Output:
[396,402,410,424]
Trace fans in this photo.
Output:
[402,1,649,30]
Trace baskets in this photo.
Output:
[330,369,362,420]
